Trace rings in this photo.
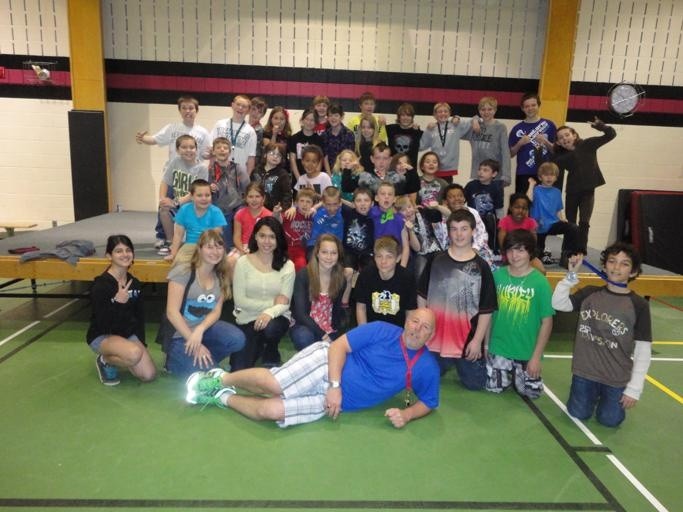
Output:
[258,320,263,326]
[327,404,331,408]
[202,355,206,359]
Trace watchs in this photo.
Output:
[327,381,342,388]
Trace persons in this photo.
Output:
[135,92,618,292]
[352,237,418,327]
[85,234,156,386]
[229,217,296,374]
[185,308,441,430]
[418,208,498,390]
[289,233,353,351]
[484,229,556,399]
[163,229,246,380]
[552,241,652,426]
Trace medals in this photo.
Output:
[403,395,413,408]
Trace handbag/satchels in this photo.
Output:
[154,311,176,345]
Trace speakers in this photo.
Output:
[618,189,683,275]
[68,110,108,223]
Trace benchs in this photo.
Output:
[0,223,38,239]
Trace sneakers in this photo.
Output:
[185,385,237,412]
[157,242,171,256]
[95,354,120,386]
[154,237,165,248]
[186,368,228,393]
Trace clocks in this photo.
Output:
[607,83,641,118]
